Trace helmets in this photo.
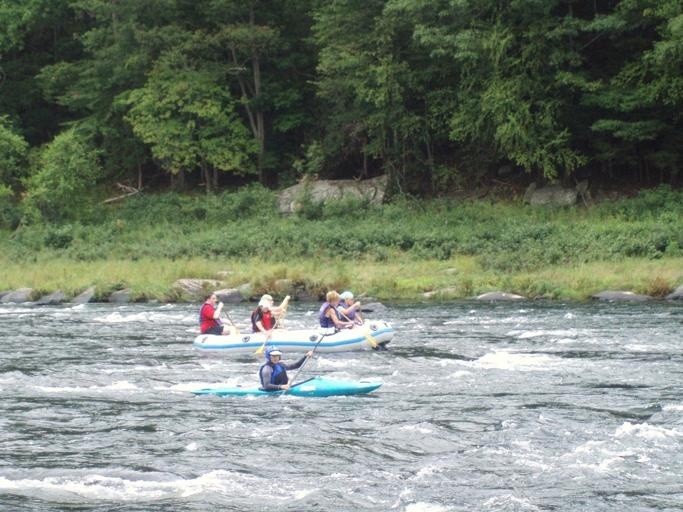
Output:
[340,292,353,300]
[266,346,281,361]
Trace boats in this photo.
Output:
[191,319,395,355]
[189,375,384,396]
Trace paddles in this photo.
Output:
[253,295,290,353]
[335,306,377,348]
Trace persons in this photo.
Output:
[200,293,238,336]
[260,347,313,390]
[251,294,291,338]
[320,290,364,336]
